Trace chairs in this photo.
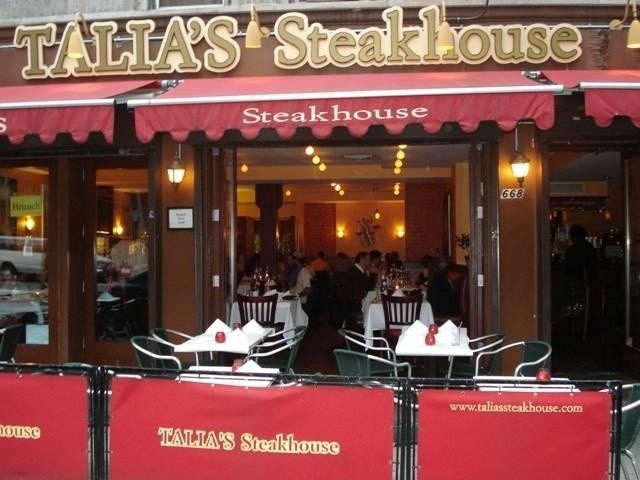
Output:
[0,322,25,379]
[553,253,608,344]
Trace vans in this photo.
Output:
[1,234,115,283]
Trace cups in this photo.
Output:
[450,325,461,346]
[534,366,554,381]
[230,322,243,334]
[231,358,249,374]
[424,323,439,346]
[215,331,227,344]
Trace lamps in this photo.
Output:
[509,148,531,189]
[166,152,185,189]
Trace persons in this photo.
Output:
[0,261,50,370]
[236,250,451,317]
[109,238,149,331]
[569,225,601,344]
